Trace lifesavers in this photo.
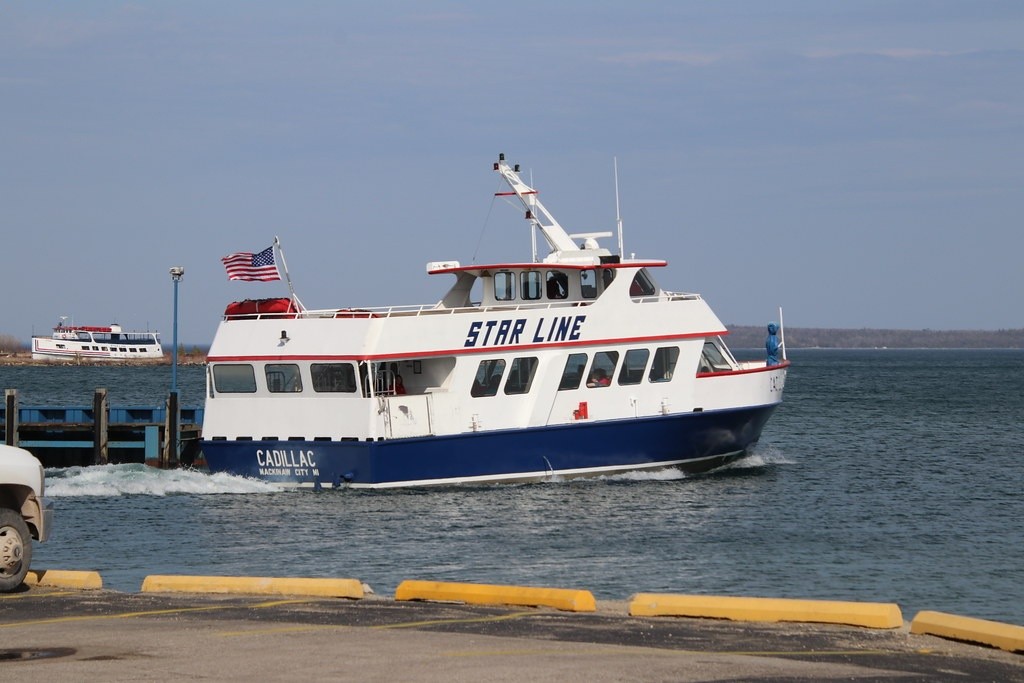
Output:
[62,334,66,339]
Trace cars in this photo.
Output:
[1,443,46,592]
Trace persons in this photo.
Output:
[385,376,405,396]
[546,277,562,300]
[629,282,642,296]
[766,322,784,367]
[586,368,611,387]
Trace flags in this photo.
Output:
[221,244,282,283]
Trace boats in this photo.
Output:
[198,154,792,489]
[31,315,164,361]
